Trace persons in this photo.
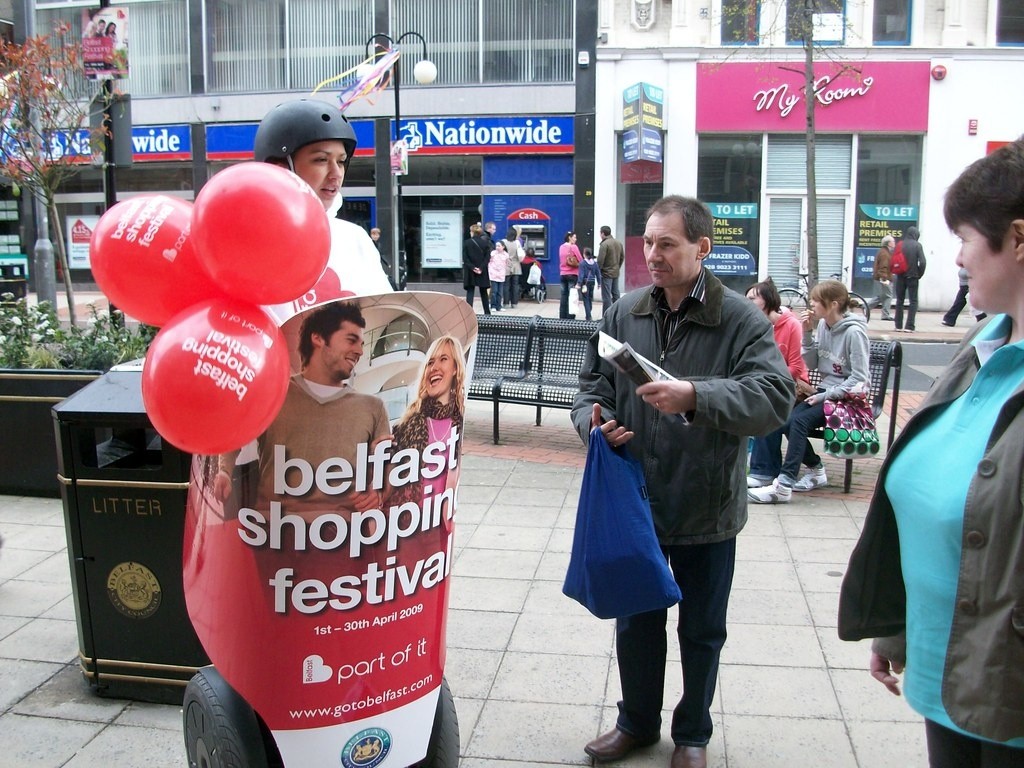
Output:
[895,226,926,333]
[747,280,870,504]
[743,282,810,487]
[214,303,465,603]
[255,98,394,326]
[597,226,625,315]
[83,19,118,47]
[559,231,583,319]
[503,225,525,309]
[571,195,796,768]
[482,221,496,244]
[488,240,511,311]
[370,227,391,268]
[861,234,895,321]
[462,224,491,314]
[521,247,546,290]
[837,135,1024,768]
[576,247,602,320]
[942,267,987,327]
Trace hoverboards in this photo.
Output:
[182,666,461,768]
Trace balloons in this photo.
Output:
[88,161,331,457]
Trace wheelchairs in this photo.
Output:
[518,259,547,304]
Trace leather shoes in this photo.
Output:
[670,745,707,768]
[584,728,660,761]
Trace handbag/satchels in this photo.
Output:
[514,241,526,261]
[793,378,818,402]
[568,287,579,315]
[966,291,983,316]
[527,264,541,285]
[566,242,579,267]
[823,386,879,459]
[891,241,908,274]
[561,427,682,618]
[581,284,587,293]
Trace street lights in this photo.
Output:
[364,32,438,295]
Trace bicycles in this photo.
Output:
[778,273,871,324]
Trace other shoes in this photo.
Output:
[492,307,496,311]
[862,303,867,316]
[896,328,902,331]
[511,304,516,307]
[904,328,915,333]
[942,321,953,327]
[882,316,896,321]
[503,304,509,308]
[500,307,506,311]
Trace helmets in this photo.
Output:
[254,99,357,163]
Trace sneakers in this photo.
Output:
[747,477,791,503]
[793,465,827,492]
[746,476,775,487]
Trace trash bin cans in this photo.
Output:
[51,358,213,708]
[0,264,27,309]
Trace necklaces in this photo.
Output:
[429,417,452,441]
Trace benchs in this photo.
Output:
[464,315,902,495]
